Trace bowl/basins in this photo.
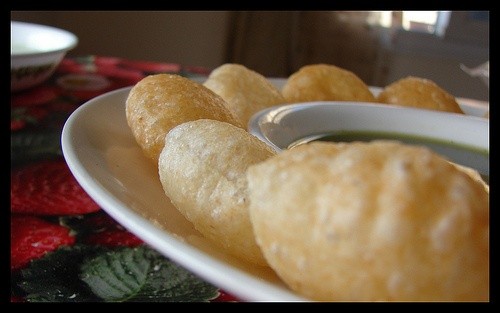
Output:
[10,19,78,93]
[246,101,490,184]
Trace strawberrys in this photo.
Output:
[10,57,241,301]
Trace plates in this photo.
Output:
[60,77,490,303]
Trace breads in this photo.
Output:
[126,64,488,302]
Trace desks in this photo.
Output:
[12,54,254,302]
